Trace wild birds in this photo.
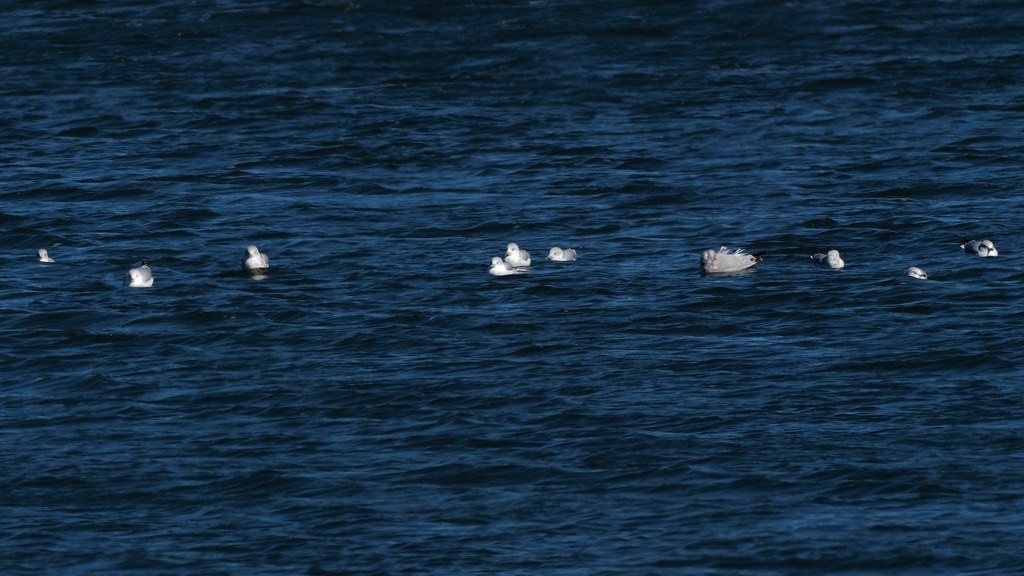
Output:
[240,245,271,270]
[809,249,844,268]
[545,247,578,261]
[487,242,530,275]
[904,266,927,280]
[123,260,153,287]
[700,246,766,273]
[35,247,55,263]
[961,238,999,257]
